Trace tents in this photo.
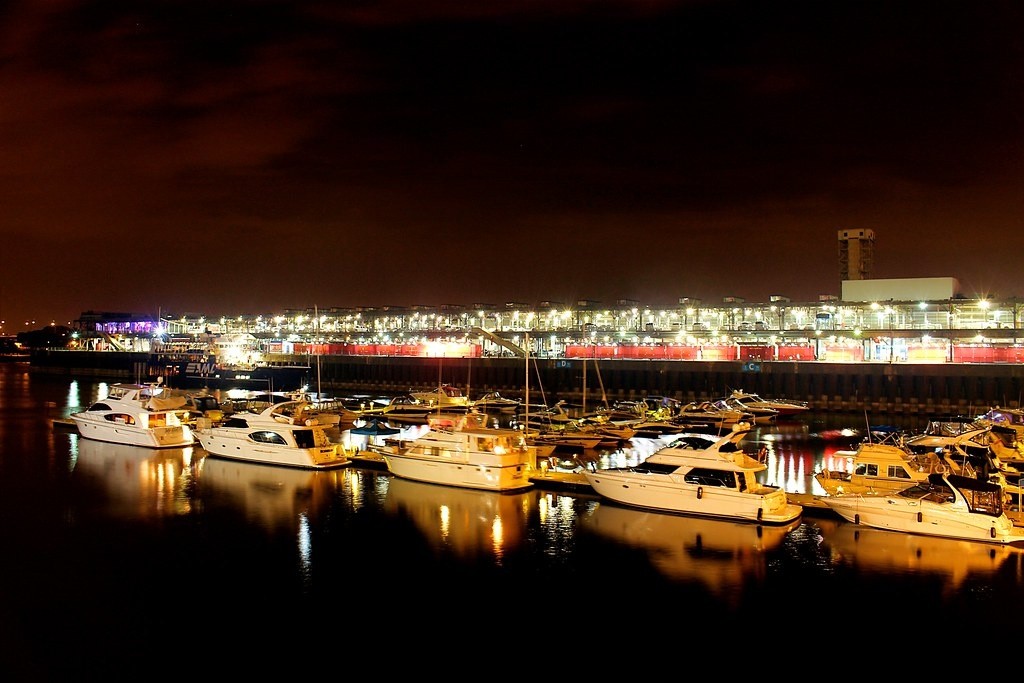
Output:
[350,418,400,453]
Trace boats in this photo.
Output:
[382,422,537,492]
[69,389,200,448]
[191,399,353,469]
[582,421,804,523]
[814,402,1024,546]
[107,346,812,459]
